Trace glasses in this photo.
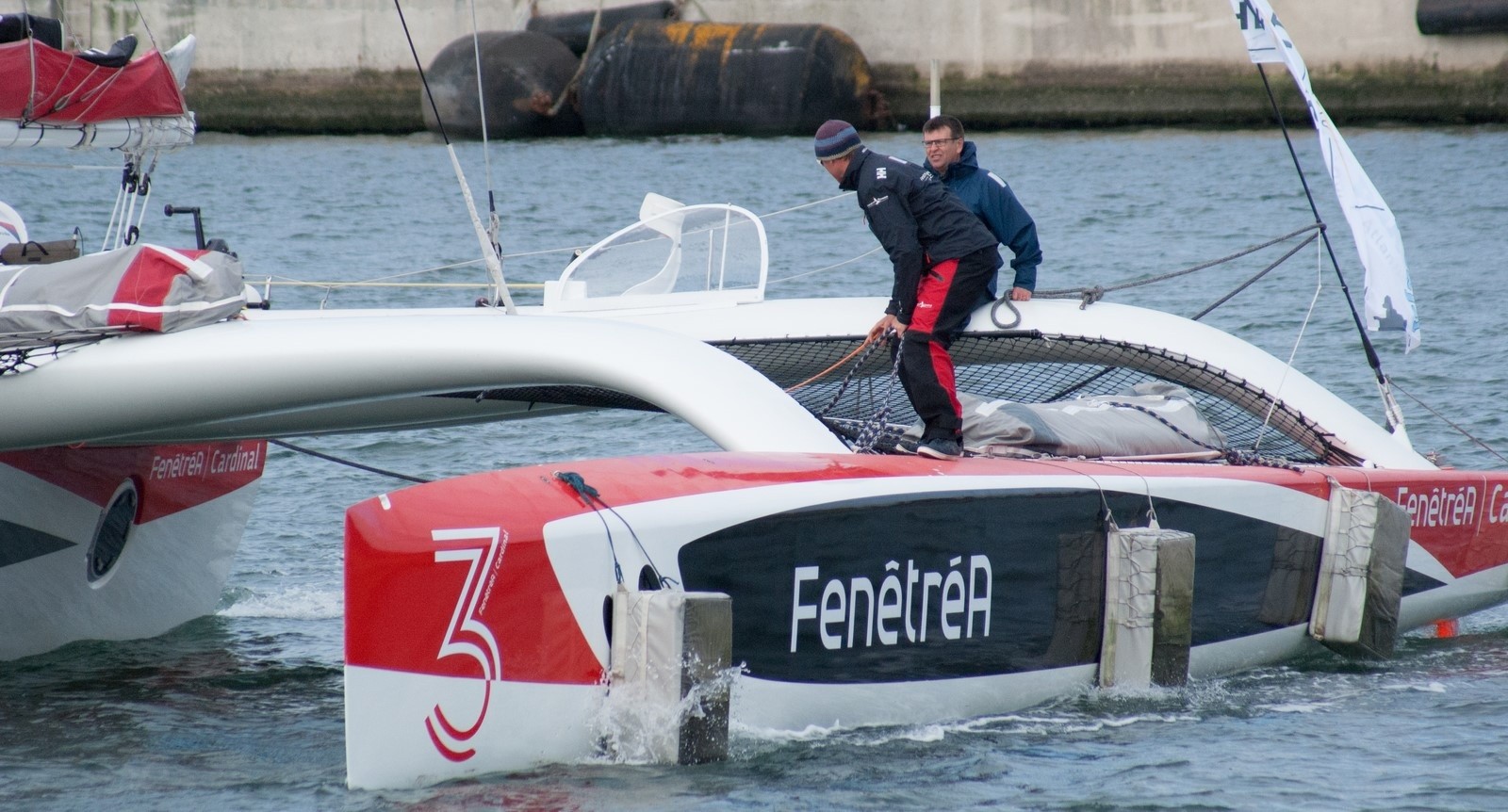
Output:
[921,138,958,148]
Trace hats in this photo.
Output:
[815,119,862,160]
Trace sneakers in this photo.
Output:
[895,435,964,461]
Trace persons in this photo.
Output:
[921,116,1043,302]
[815,119,1005,460]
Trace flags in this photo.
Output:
[1229,0,1421,357]
